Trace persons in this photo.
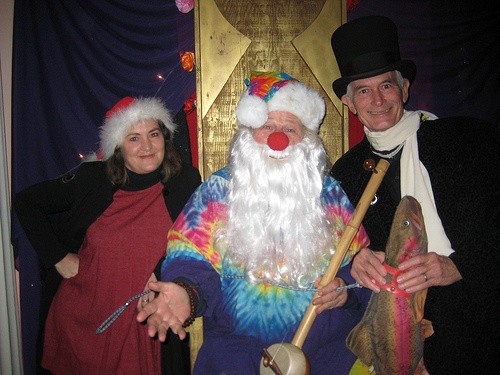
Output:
[320,21,499,374]
[137,71,371,375]
[18,97,201,375]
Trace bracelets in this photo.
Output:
[175,282,196,328]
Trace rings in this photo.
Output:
[142,299,149,302]
[423,274,428,281]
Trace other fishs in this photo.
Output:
[345,195,435,375]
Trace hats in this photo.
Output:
[235,69,325,133]
[99,95,178,161]
[331,13,416,101]
